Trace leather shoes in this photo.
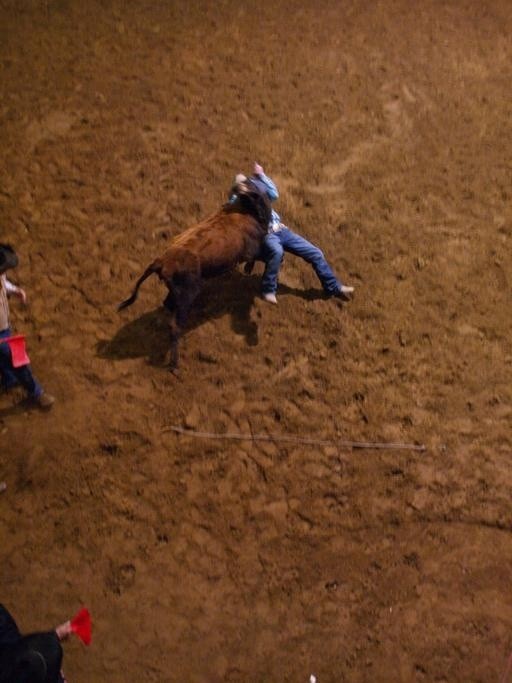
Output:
[38,392,55,408]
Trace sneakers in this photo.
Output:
[264,291,277,304]
[340,285,354,294]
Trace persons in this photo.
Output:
[1,241,56,408]
[0,601,93,682]
[229,161,353,302]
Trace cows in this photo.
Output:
[115,182,272,373]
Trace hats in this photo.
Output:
[0,244,18,274]
